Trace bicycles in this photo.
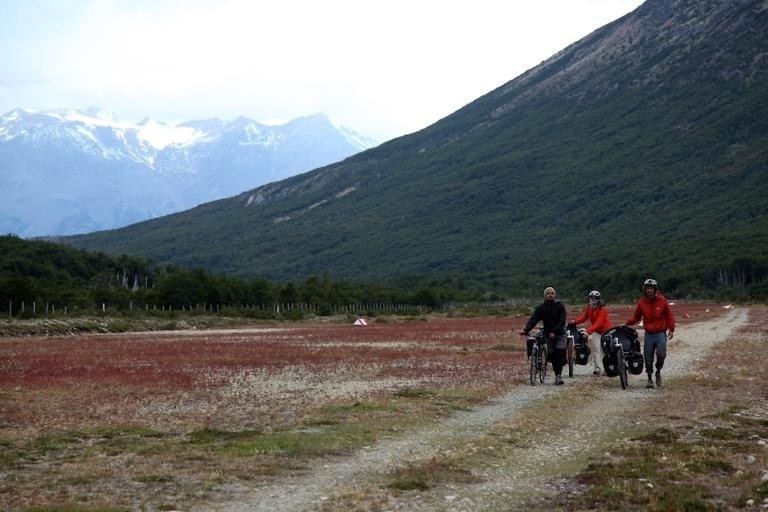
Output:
[517,315,645,391]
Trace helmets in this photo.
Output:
[589,291,600,297]
[643,279,658,295]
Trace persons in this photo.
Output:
[619,278,676,389]
[568,290,614,376]
[519,286,567,385]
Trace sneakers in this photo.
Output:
[646,380,653,388]
[655,371,661,386]
[554,375,564,385]
[593,368,600,375]
